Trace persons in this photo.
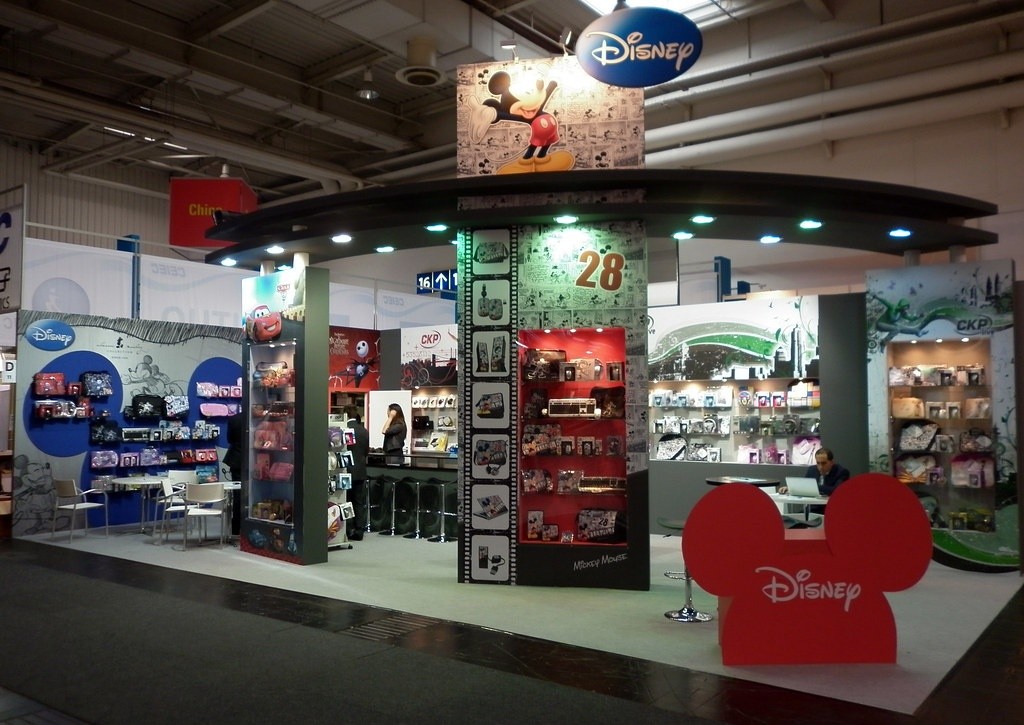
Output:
[777,448,850,530]
[382,403,407,465]
[343,404,370,541]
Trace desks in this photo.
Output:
[112,476,167,538]
[770,493,829,520]
[195,481,242,547]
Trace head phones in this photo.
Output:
[704,419,717,433]
[783,419,798,434]
[486,451,504,475]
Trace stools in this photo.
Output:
[783,514,822,529]
[658,517,713,622]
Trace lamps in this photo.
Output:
[558,26,572,57]
[356,71,380,99]
[500,40,519,62]
[737,281,766,295]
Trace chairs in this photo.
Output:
[52,477,109,543]
[147,471,228,550]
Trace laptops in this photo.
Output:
[786,477,828,499]
[473,495,508,520]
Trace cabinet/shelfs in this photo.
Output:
[890,340,995,483]
[704,476,780,490]
[518,328,626,545]
[732,377,820,465]
[648,379,732,466]
[250,343,295,529]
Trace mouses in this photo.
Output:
[335,434,351,518]
[39,386,240,466]
[653,392,785,465]
[928,372,994,530]
[560,364,620,456]
